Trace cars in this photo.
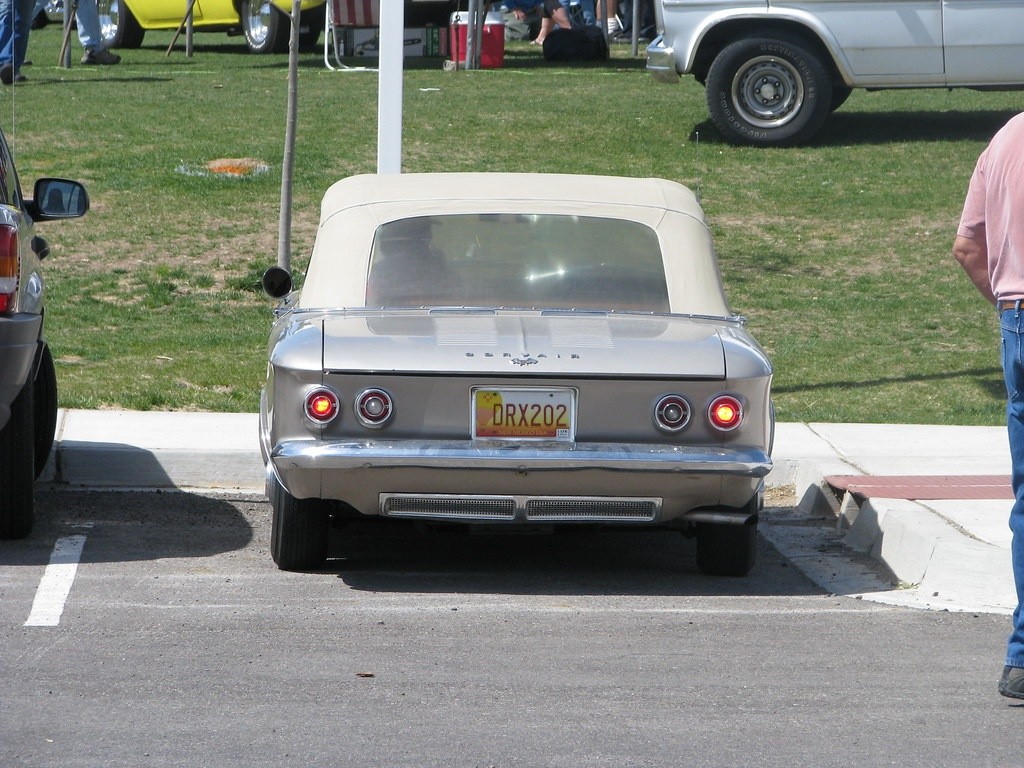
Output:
[259,171,774,577]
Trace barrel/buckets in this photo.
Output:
[449,9,507,71]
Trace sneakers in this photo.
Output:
[80,47,121,64]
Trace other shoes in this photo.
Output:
[0,63,26,84]
[1000,666,1024,708]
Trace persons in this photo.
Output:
[31,0,121,66]
[951,108,1024,701]
[0,0,33,81]
[493,0,656,49]
